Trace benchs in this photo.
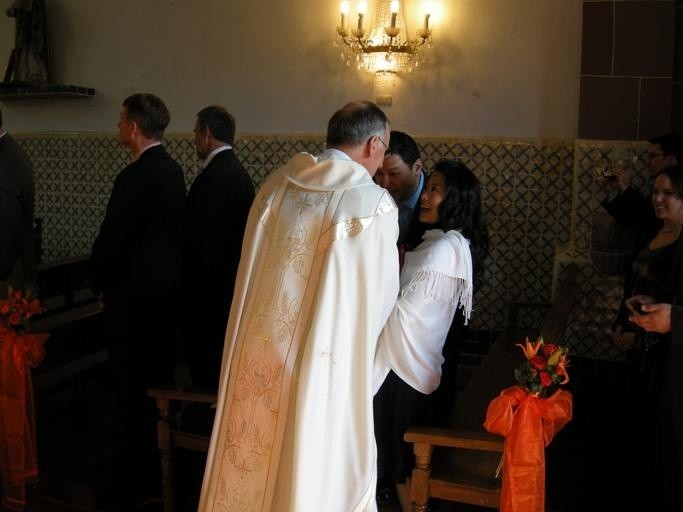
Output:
[148,387,218,512]
[402,276,577,512]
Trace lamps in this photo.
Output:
[336,0,433,105]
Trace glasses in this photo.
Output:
[379,138,393,160]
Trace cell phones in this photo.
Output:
[630,297,647,314]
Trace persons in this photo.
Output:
[185,105,255,421]
[194,97,445,511]
[630,165,683,512]
[378,156,489,493]
[623,234,683,511]
[88,91,188,490]
[375,131,484,355]
[597,132,683,343]
[0,110,36,295]
